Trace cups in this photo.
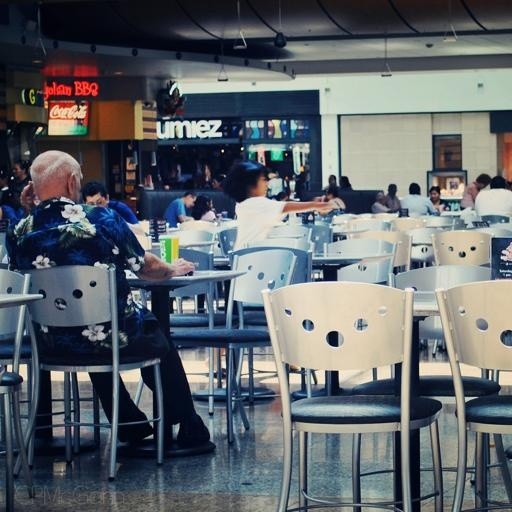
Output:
[216,214,222,219]
[221,211,228,218]
[159,234,181,266]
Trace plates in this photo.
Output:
[222,218,232,221]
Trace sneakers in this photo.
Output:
[128,423,152,442]
[177,415,210,441]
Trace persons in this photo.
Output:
[206,159,347,378]
[261,167,512,226]
[5,150,210,447]
[0,158,235,231]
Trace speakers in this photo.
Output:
[489,110,512,134]
